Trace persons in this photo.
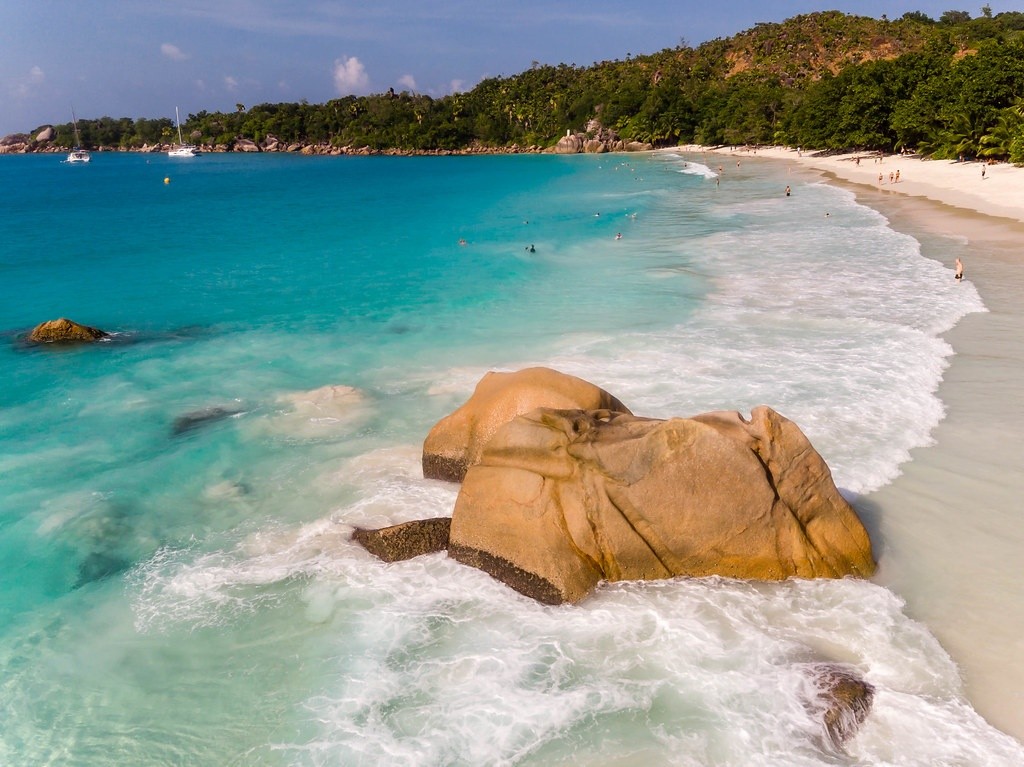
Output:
[900,146,905,157]
[785,186,791,196]
[874,152,883,164]
[597,212,599,215]
[955,258,964,282]
[879,172,883,183]
[530,244,535,253]
[889,169,900,184]
[592,142,784,221]
[981,164,987,180]
[855,155,861,165]
[797,147,801,154]
[617,232,621,238]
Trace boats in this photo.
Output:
[167,106,198,157]
[65,107,93,164]
